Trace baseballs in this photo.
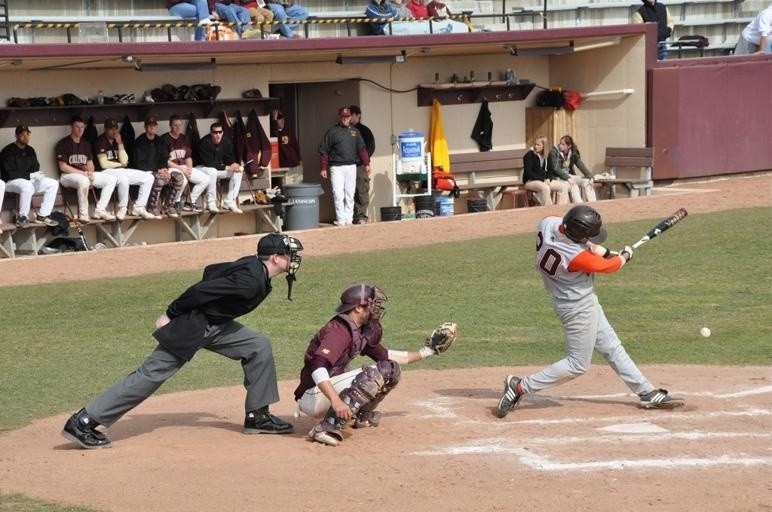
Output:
[700,327,711,338]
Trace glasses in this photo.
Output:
[213,130,223,134]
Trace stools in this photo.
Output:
[510,182,602,209]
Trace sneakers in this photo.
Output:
[114,94,136,103]
[496,371,524,419]
[77,209,91,223]
[185,202,204,213]
[92,207,129,220]
[244,413,294,436]
[60,407,113,452]
[222,199,244,214]
[352,419,379,428]
[206,201,220,215]
[638,388,686,411]
[15,214,28,230]
[35,216,60,228]
[132,207,163,220]
[164,205,180,217]
[306,422,342,445]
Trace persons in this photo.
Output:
[194,123,244,214]
[366,0,452,34]
[631,1,673,61]
[92,118,155,220]
[164,0,309,41]
[160,113,209,212]
[317,108,370,226]
[733,1,772,56]
[59,232,302,450]
[269,108,304,185]
[131,116,188,215]
[54,117,118,223]
[549,135,596,204]
[520,135,568,206]
[348,105,375,225]
[294,284,456,447]
[497,205,686,418]
[0,123,58,227]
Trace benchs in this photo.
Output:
[0,166,294,257]
[592,146,655,197]
[420,147,530,191]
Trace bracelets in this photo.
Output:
[419,345,436,360]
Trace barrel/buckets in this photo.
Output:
[434,195,454,216]
[467,199,487,213]
[413,196,436,219]
[282,184,321,231]
[396,132,426,175]
[381,207,401,221]
[269,137,280,169]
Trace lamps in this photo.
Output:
[395,48,430,64]
[573,34,621,53]
[508,45,522,56]
[335,55,344,64]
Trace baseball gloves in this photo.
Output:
[423,322,457,356]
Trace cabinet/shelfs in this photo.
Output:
[526,106,576,156]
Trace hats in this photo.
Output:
[144,115,158,125]
[14,123,33,134]
[102,119,121,128]
[338,106,353,118]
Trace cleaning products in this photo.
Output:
[505,69,513,83]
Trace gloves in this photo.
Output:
[618,245,634,261]
[603,247,620,260]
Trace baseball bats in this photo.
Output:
[631,208,688,249]
[66,200,91,251]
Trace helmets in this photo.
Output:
[257,233,304,277]
[334,283,387,322]
[561,205,609,247]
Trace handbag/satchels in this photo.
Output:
[669,34,710,48]
[431,165,461,199]
